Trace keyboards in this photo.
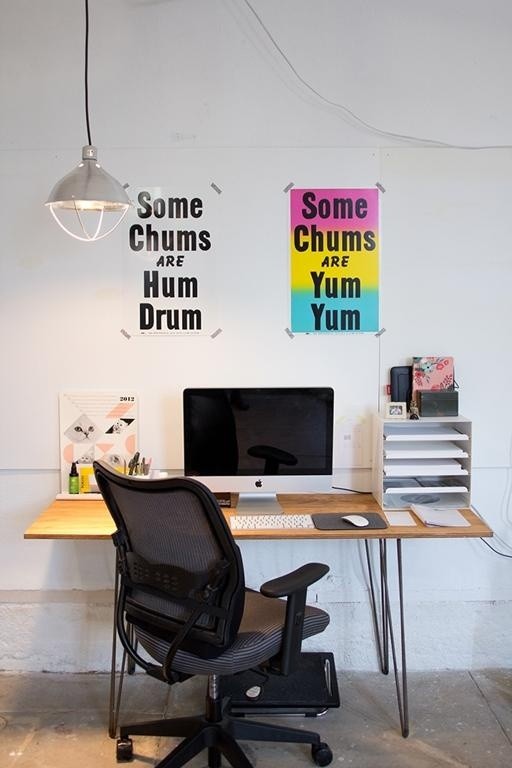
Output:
[229,514,316,531]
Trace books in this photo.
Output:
[409,502,472,528]
[412,355,455,406]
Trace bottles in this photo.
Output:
[68,462,79,494]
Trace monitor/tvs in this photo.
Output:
[182,388,334,513]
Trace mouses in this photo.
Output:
[341,515,369,527]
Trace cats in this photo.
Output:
[63,414,104,444]
[78,450,95,464]
[112,418,128,434]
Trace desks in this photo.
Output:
[20,491,494,740]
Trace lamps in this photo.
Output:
[44,2,134,245]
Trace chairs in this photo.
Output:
[91,461,335,768]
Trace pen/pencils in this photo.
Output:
[128,451,152,477]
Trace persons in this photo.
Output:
[391,406,402,414]
